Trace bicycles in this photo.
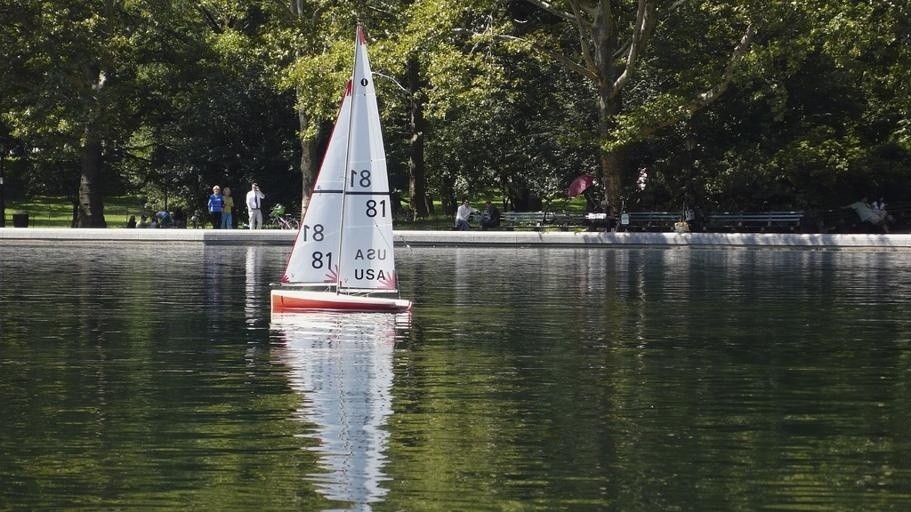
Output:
[265,208,299,230]
[542,195,568,225]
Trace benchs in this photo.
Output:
[465,200,910,231]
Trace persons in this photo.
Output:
[479,202,500,230]
[220,187,234,228]
[128,210,175,228]
[591,201,616,232]
[840,195,896,233]
[207,185,226,229]
[455,198,480,231]
[245,182,265,229]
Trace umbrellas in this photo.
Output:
[567,175,594,197]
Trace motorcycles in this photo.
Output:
[605,195,629,231]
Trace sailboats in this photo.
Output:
[270,18,412,313]
